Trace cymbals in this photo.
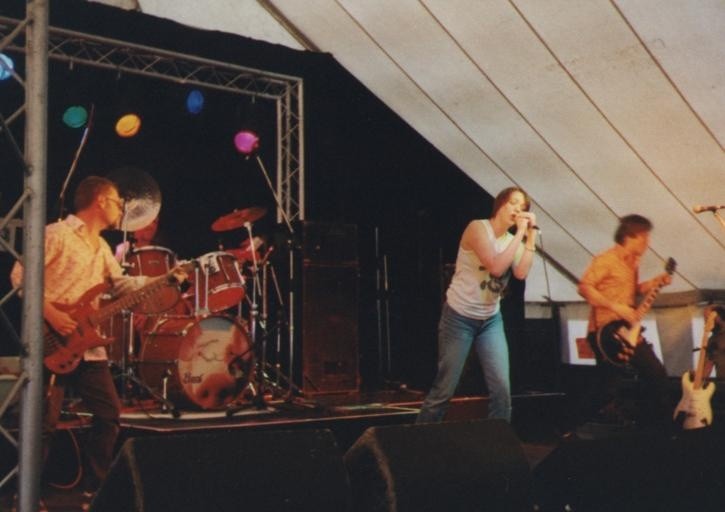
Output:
[104,168,161,233]
[225,249,259,262]
[211,207,268,231]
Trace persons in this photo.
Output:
[9,175,188,509]
[413,187,537,428]
[569,215,674,429]
[697,304,724,386]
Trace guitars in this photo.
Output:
[43,258,198,374]
[671,310,717,430]
[596,257,675,367]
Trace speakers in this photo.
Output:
[89,430,346,512]
[343,419,521,512]
[268,221,359,264]
[268,264,360,396]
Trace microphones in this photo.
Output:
[513,214,538,231]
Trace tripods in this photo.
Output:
[69,319,180,419]
[226,312,303,415]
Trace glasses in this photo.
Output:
[107,198,125,209]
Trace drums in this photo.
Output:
[124,247,180,314]
[185,252,247,314]
[138,317,256,411]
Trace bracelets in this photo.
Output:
[524,243,536,253]
